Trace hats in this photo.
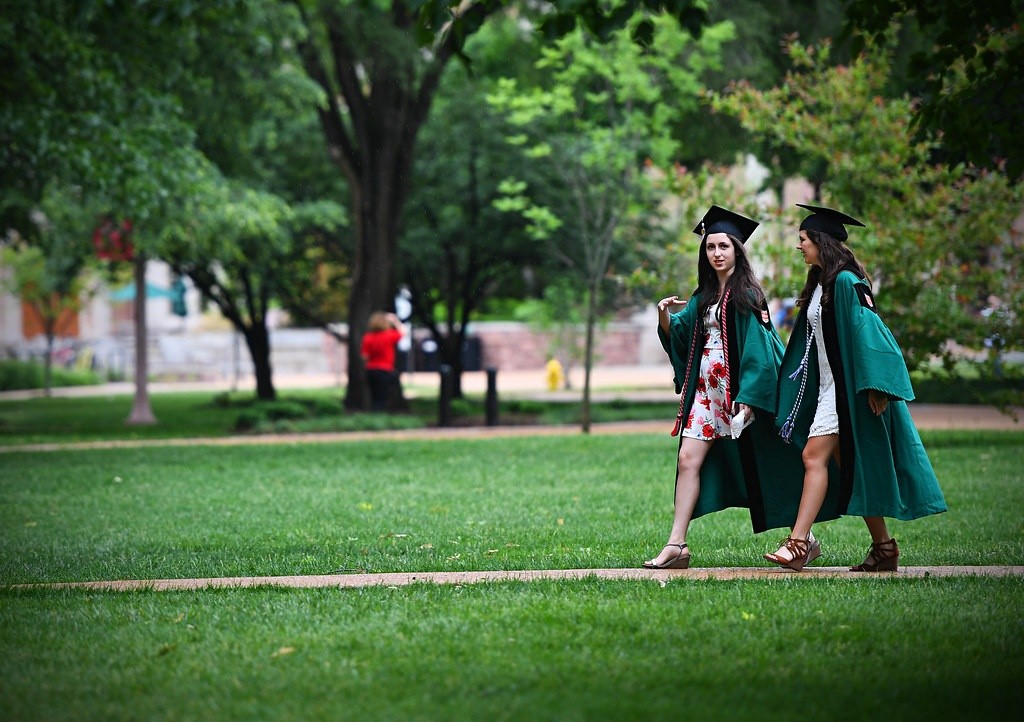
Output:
[693,205,760,246]
[797,203,867,242]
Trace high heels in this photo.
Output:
[763,537,812,572]
[850,538,900,572]
[643,544,691,569]
[803,541,822,565]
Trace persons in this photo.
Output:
[359,310,406,415]
[642,203,824,570]
[543,352,565,394]
[763,205,948,571]
